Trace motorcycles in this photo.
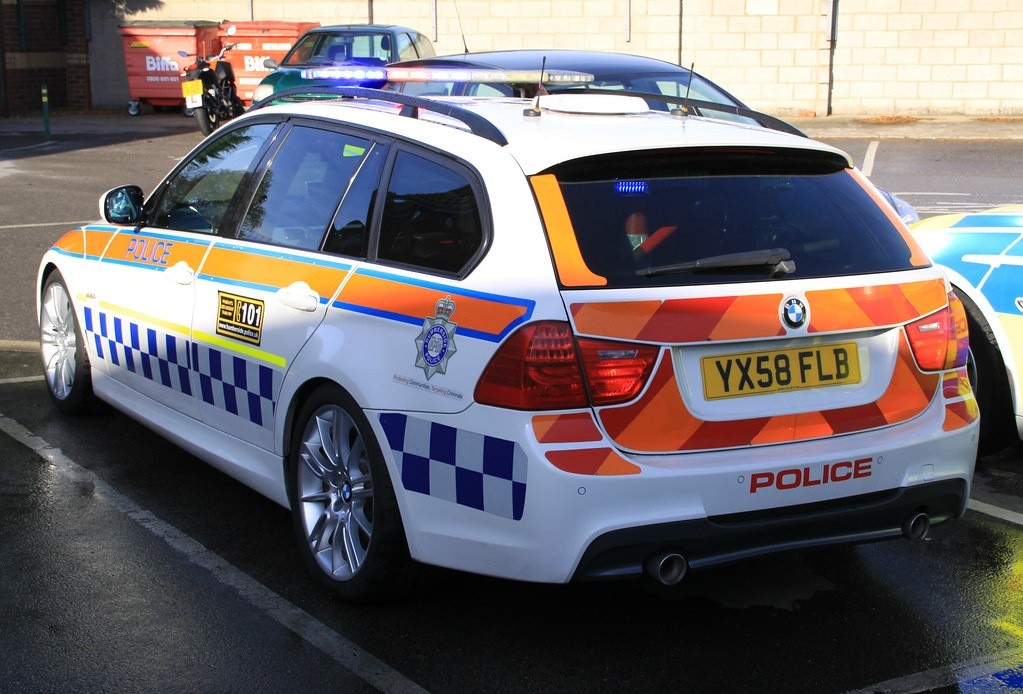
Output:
[177,42,246,137]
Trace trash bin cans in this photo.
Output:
[216,21,321,108]
[116,20,221,118]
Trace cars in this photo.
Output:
[907,203,1023,443]
[252,25,437,106]
[353,49,920,259]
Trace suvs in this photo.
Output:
[36,65,982,602]
